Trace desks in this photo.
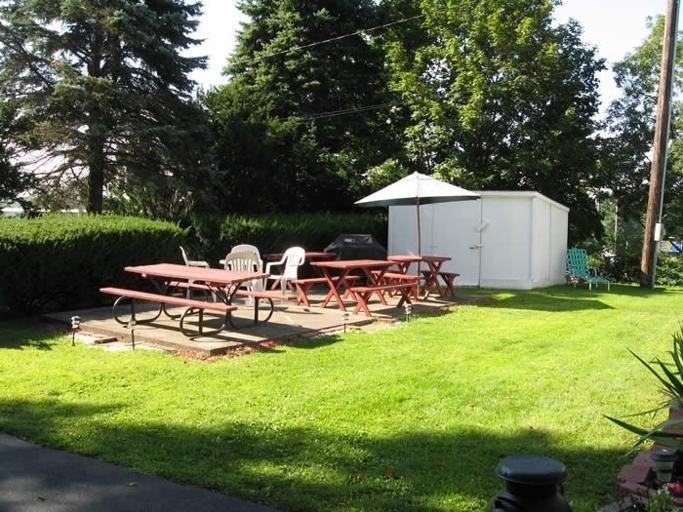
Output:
[264,251,453,315]
[124,262,270,337]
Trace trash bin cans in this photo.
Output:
[339,233,374,283]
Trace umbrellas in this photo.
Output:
[354,169,482,282]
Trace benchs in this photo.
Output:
[98,285,237,337]
[165,280,298,328]
[291,272,459,316]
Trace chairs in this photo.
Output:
[180,242,305,304]
[566,249,610,292]
